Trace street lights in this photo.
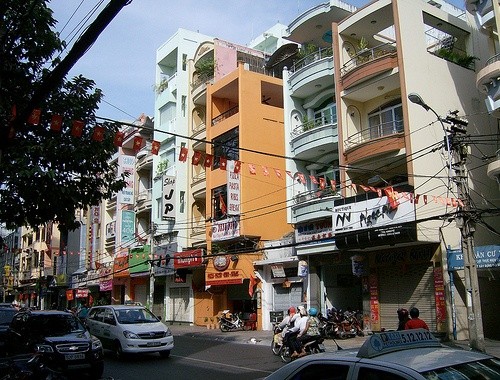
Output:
[408,92,485,350]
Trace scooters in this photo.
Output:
[218,312,248,332]
[271,308,366,363]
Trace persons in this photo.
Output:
[274,306,322,358]
[396,307,429,331]
[13,300,91,322]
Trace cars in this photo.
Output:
[0,303,19,333]
[254,328,500,380]
[0,326,54,380]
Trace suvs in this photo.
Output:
[8,307,105,380]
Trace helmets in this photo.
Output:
[297,304,307,316]
[309,307,318,315]
[396,308,408,320]
[288,306,296,314]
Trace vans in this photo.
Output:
[82,304,175,362]
